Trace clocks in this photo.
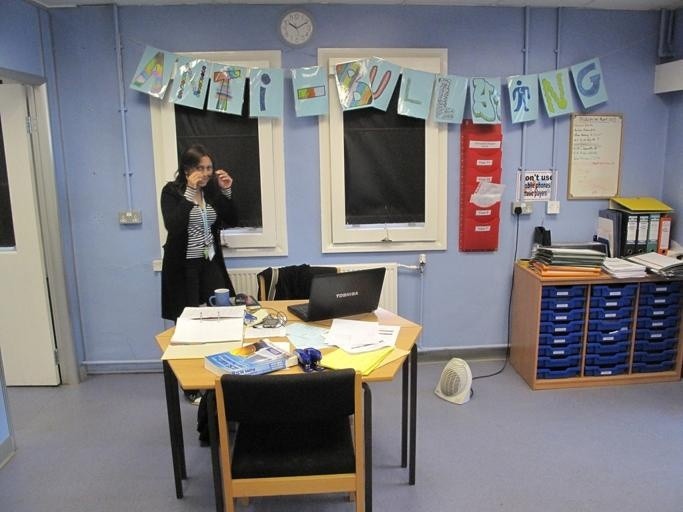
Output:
[277,7,316,49]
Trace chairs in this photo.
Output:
[206,366,372,512]
[256,263,341,301]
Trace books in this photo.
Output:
[526,195,682,279]
[201,337,299,380]
[168,304,247,345]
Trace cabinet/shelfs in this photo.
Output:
[509,262,683,390]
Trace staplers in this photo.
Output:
[293,350,322,372]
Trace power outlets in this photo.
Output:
[510,201,522,215]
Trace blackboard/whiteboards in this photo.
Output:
[566,112,624,201]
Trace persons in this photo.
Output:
[158,140,237,407]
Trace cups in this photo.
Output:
[209,289,229,306]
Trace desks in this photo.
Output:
[153,298,424,499]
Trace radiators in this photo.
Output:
[225,263,398,315]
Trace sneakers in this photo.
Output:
[183,386,205,405]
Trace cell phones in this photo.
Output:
[262,317,279,329]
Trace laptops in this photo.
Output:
[288,267,386,322]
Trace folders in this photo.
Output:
[609,198,673,256]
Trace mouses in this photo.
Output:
[235,293,247,305]
